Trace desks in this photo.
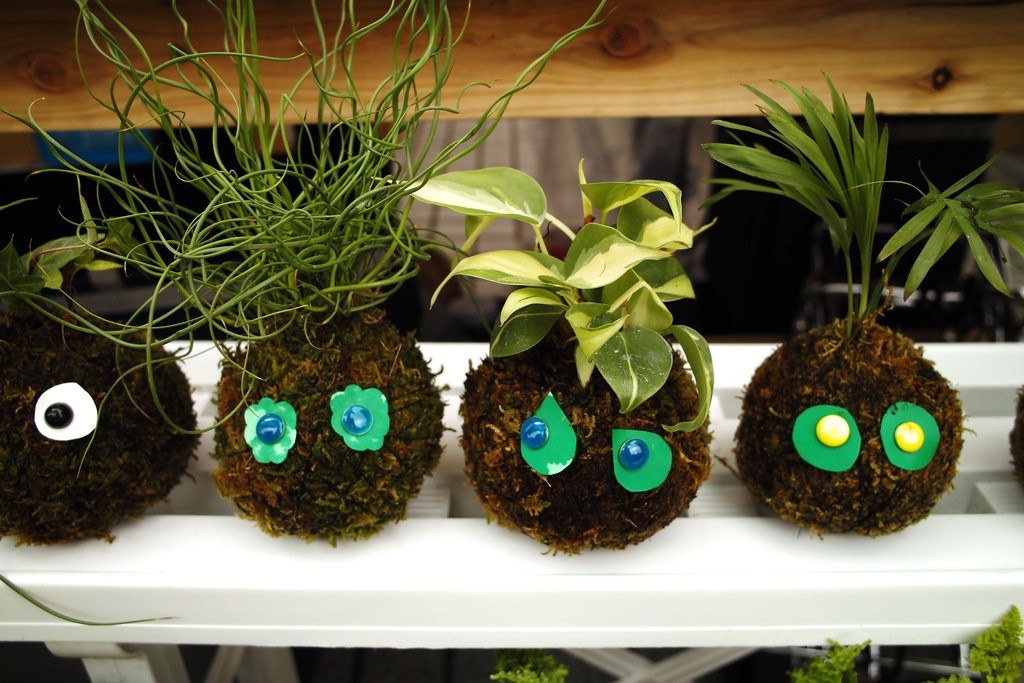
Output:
[0,340,1024,683]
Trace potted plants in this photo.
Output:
[381,156,715,553]
[692,65,1024,540]
[0,177,196,544]
[0,0,617,545]
